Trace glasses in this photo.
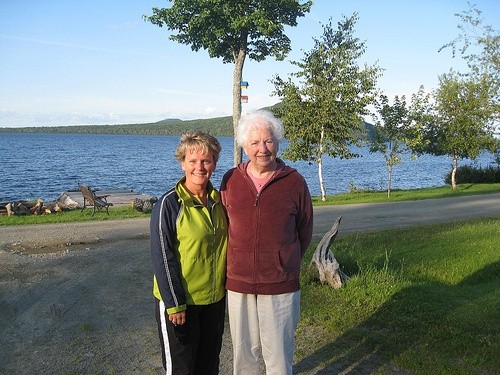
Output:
[174,324,187,345]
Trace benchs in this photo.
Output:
[79,184,114,217]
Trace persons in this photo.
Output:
[148,130,228,375]
[219,110,313,375]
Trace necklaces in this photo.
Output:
[249,164,268,187]
